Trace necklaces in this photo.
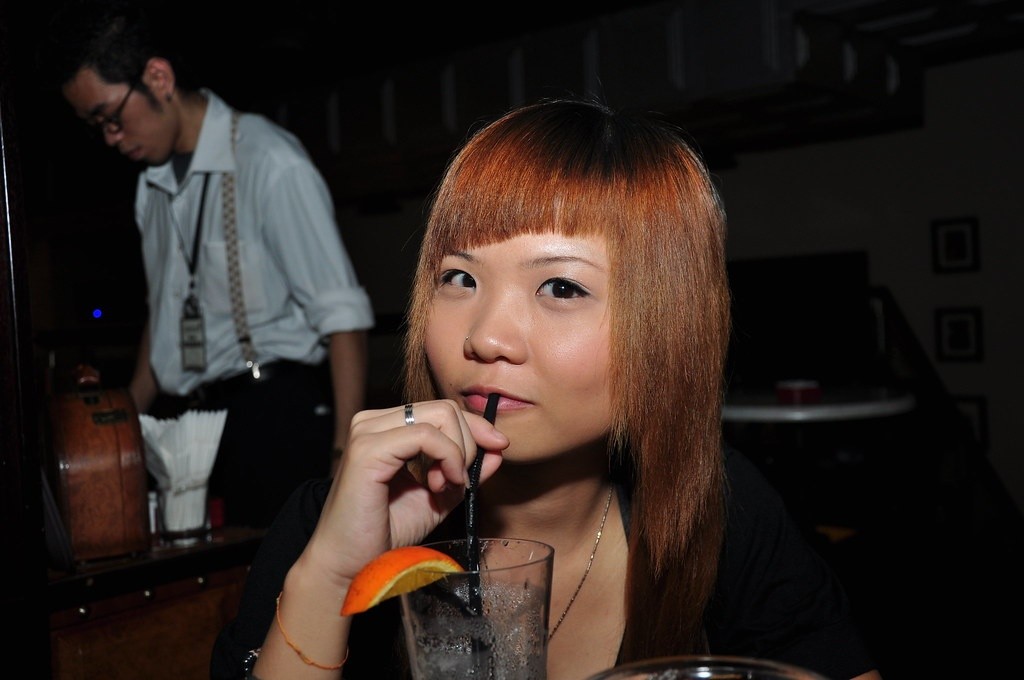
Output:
[482,481,613,642]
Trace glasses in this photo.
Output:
[82,66,148,137]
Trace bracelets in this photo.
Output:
[275,593,349,670]
[333,448,344,459]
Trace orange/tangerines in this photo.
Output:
[340,544,463,617]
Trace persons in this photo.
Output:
[46,0,376,530]
[212,99,883,680]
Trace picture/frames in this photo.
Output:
[950,393,990,455]
[929,217,981,276]
[933,306,984,363]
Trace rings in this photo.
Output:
[405,404,415,425]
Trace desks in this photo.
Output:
[719,393,913,542]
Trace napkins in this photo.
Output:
[139,407,229,545]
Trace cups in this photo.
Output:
[583,655,827,679]
[402,537,554,680]
